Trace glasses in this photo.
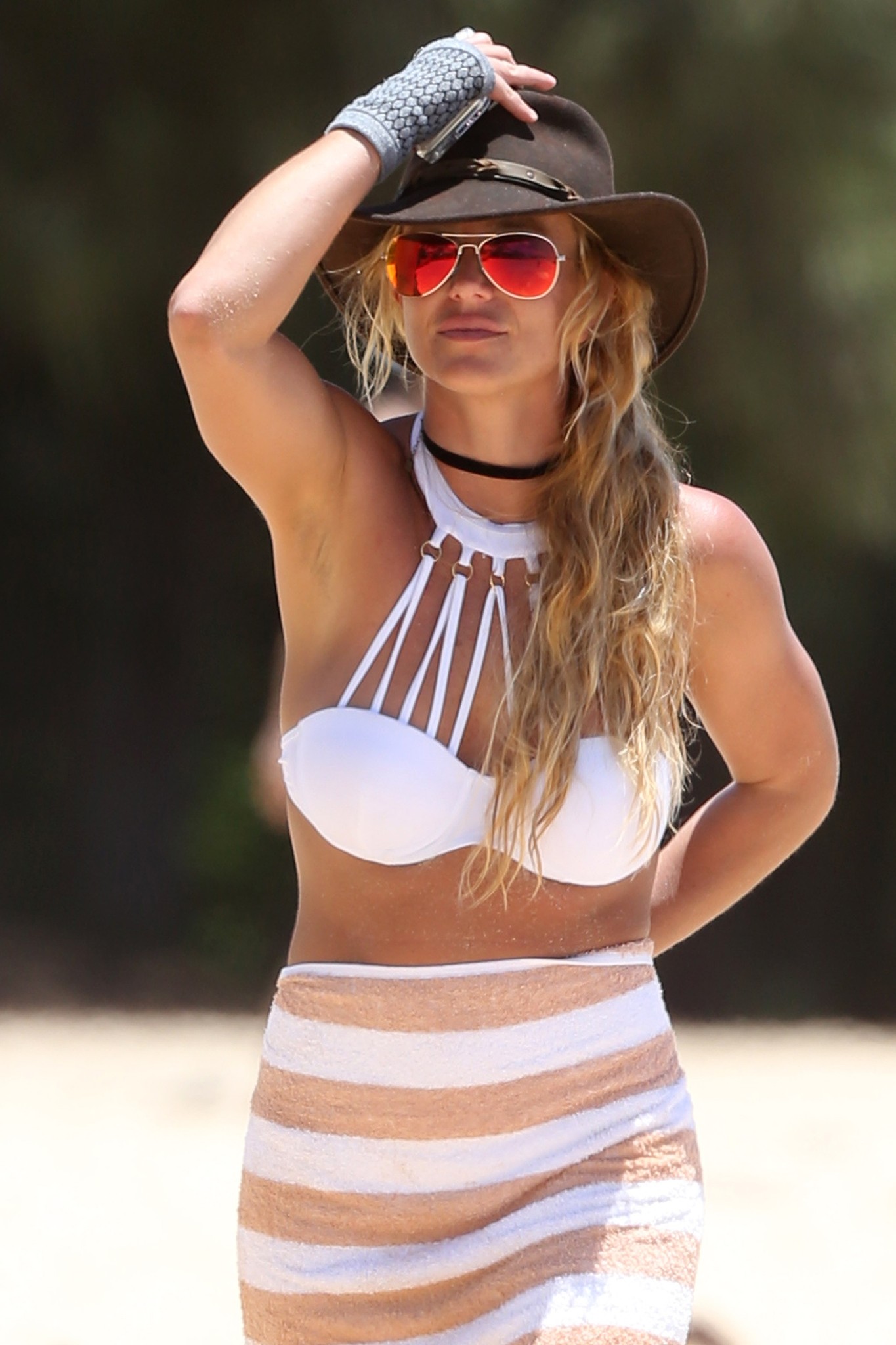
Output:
[379,231,584,300]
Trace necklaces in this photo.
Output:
[420,421,558,480]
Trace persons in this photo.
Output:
[168,28,842,1345]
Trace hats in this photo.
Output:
[314,90,708,375]
